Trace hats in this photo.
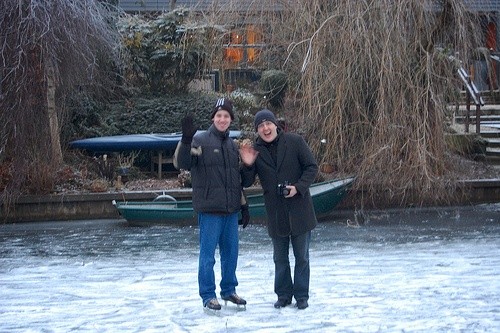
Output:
[212,96,235,120]
[254,109,280,132]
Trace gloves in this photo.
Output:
[180,111,199,145]
[238,200,250,228]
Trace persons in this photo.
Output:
[239,108,321,310]
[176,98,251,313]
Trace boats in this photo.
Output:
[65,129,255,176]
[110,175,357,226]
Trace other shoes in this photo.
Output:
[274,298,291,307]
[297,299,308,308]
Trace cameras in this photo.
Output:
[277,180,293,196]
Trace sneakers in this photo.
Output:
[222,292,246,310]
[202,296,221,316]
